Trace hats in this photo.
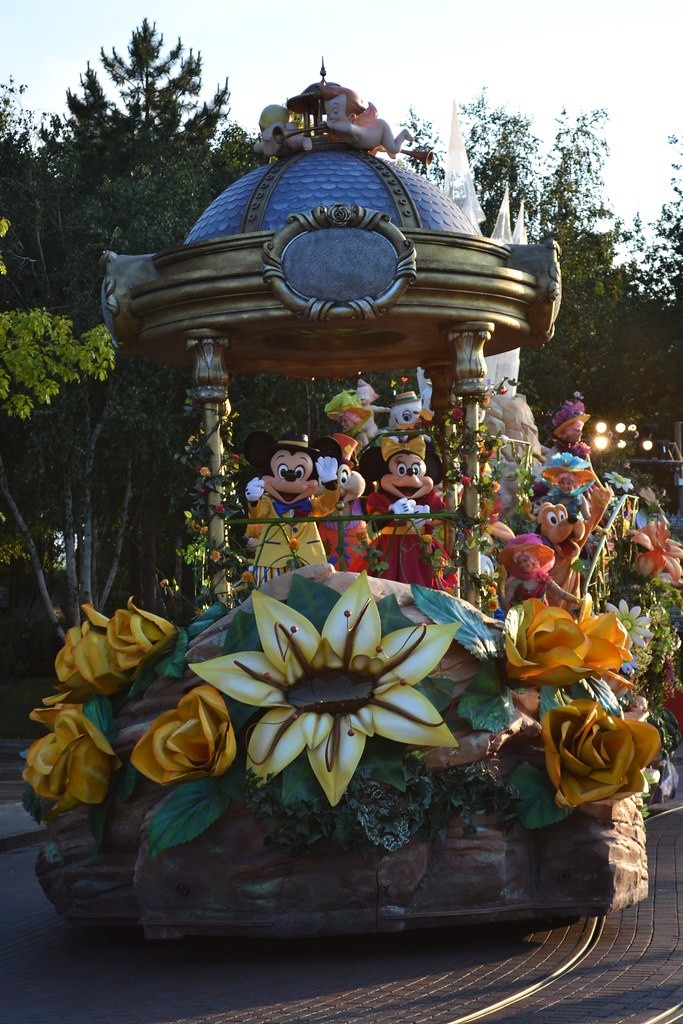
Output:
[552,391,591,436]
[323,390,373,421]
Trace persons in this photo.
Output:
[544,399,606,507]
[355,381,392,437]
[253,103,313,156]
[323,390,372,455]
[528,452,597,569]
[495,533,585,609]
[318,84,413,153]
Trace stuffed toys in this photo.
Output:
[312,435,378,577]
[387,391,435,444]
[242,433,343,590]
[491,486,611,624]
[357,438,459,592]
